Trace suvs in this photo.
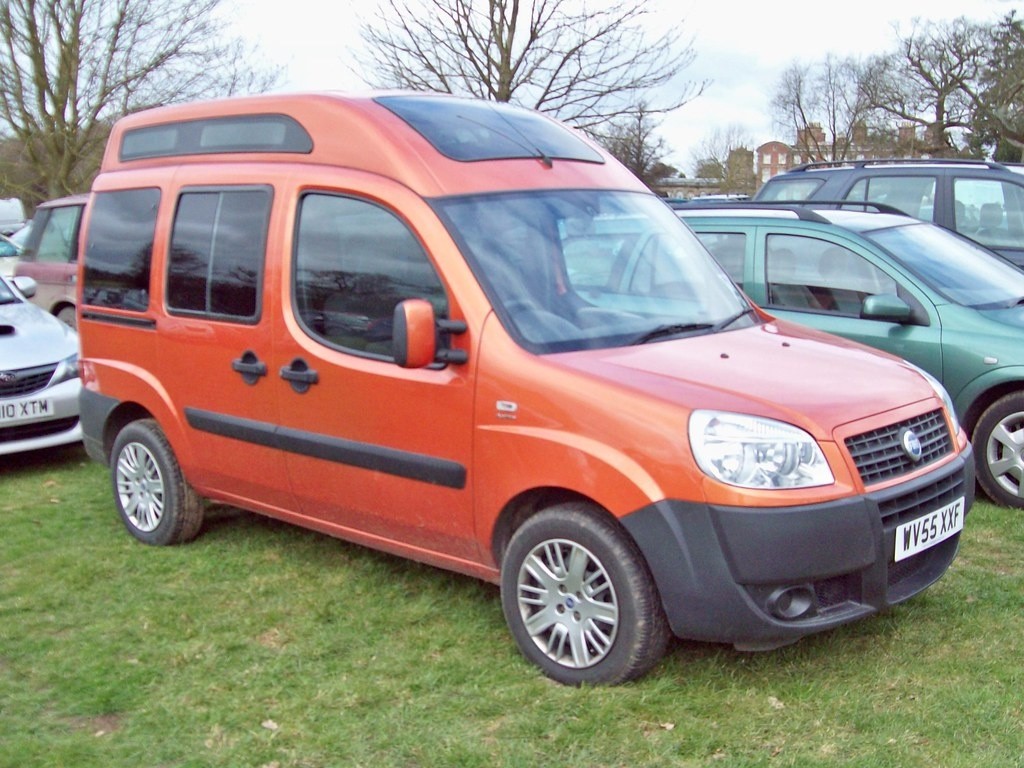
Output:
[540,200,1024,510]
[8,193,92,331]
[753,158,1023,270]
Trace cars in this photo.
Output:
[0,274,84,455]
[1,234,22,281]
[10,221,35,251]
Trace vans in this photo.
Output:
[0,198,26,238]
[75,91,976,691]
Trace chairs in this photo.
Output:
[769,249,823,312]
[976,203,1012,241]
[816,249,868,317]
[955,200,973,232]
